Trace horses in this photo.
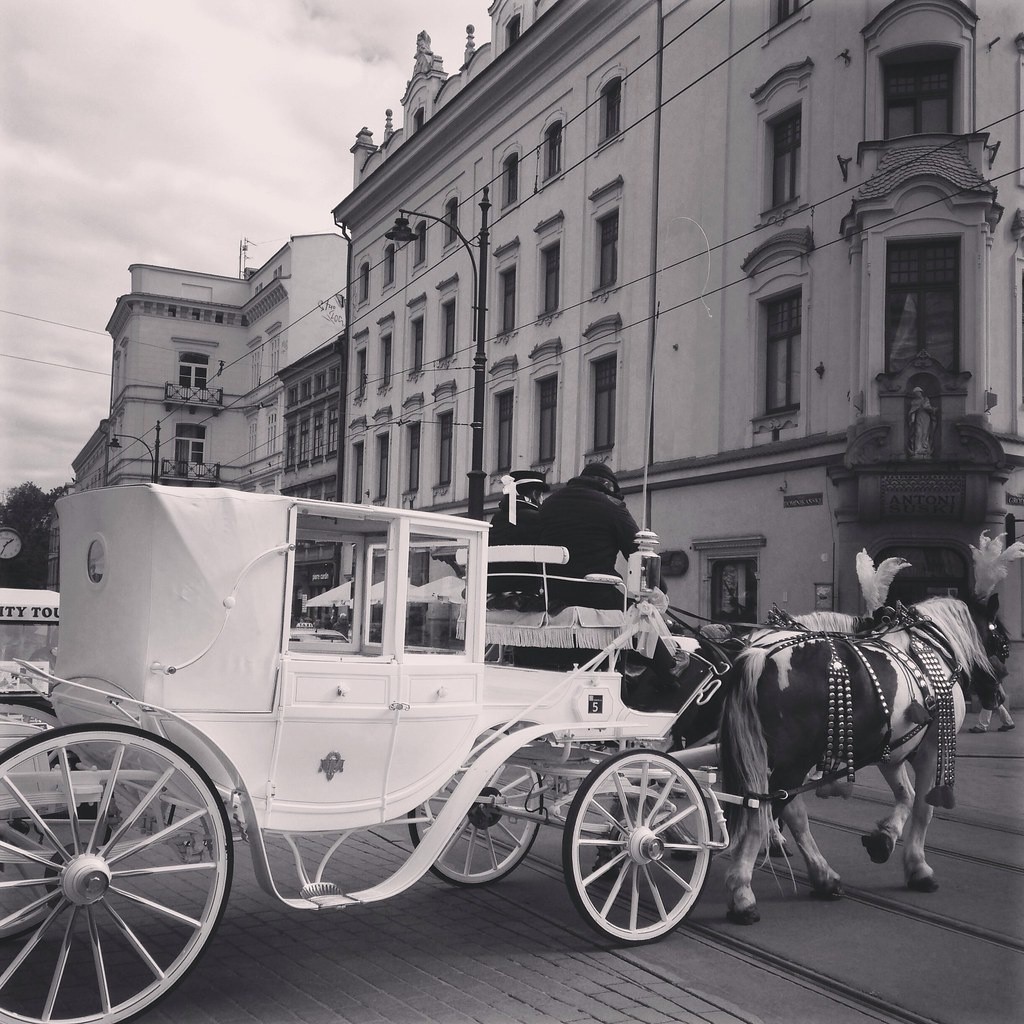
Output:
[591,587,1009,926]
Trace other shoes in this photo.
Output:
[968,727,987,733]
[998,724,1016,732]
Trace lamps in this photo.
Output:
[335,294,345,308]
[626,529,662,599]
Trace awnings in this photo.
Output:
[430,546,470,578]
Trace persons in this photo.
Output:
[528,462,692,688]
[906,385,940,461]
[1,624,59,671]
[486,470,551,595]
[291,609,352,642]
[969,616,1016,734]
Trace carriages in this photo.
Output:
[1,477,1010,1023]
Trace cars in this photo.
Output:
[290,621,350,643]
[0,587,112,845]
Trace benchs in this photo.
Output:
[454,544,677,671]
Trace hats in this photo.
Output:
[500,470,550,526]
[580,462,620,493]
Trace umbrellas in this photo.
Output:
[406,574,466,638]
[305,579,354,607]
[349,578,419,611]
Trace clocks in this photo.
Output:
[0,527,23,560]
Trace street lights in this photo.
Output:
[386,210,486,525]
[109,434,160,482]
